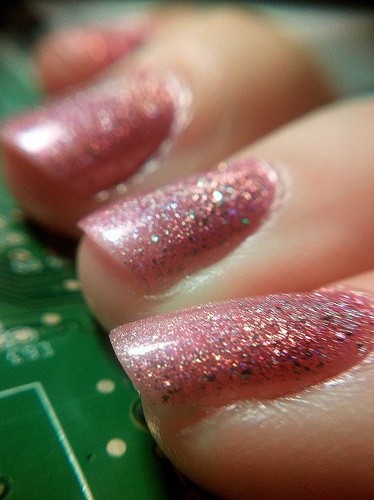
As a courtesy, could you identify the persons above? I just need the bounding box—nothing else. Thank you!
[1,7,372,500]
